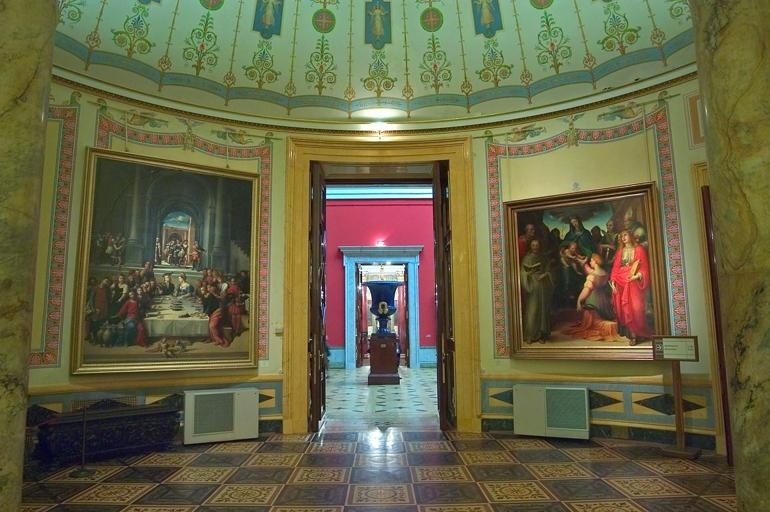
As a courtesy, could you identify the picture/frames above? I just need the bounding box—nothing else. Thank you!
[68,144,262,376]
[501,182,671,365]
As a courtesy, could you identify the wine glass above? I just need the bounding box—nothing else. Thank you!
[360,280,406,337]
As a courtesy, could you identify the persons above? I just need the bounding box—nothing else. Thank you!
[518,215,652,345]
[84,231,250,347]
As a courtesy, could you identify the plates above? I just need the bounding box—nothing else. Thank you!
[148,311,160,316]
[168,302,184,311]
